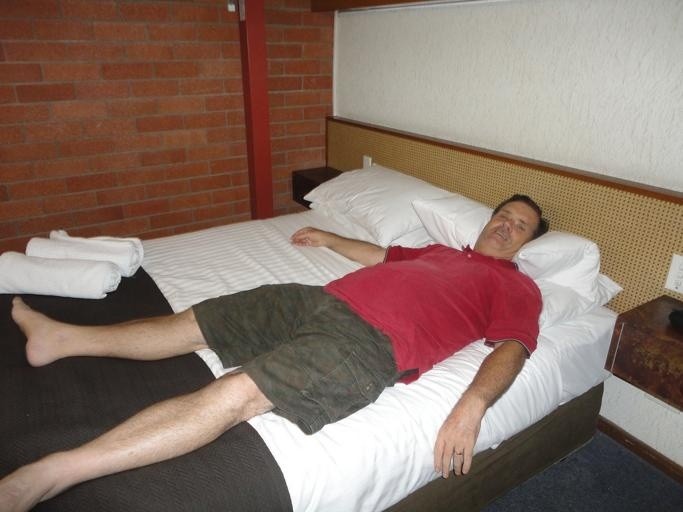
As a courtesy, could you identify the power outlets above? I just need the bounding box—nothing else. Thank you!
[362,156,372,168]
[664,253,683,295]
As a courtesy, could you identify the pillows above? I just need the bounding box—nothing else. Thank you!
[311,205,434,250]
[387,233,618,320]
[412,194,599,295]
[304,164,451,248]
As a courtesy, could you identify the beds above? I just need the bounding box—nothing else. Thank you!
[1,116,682,512]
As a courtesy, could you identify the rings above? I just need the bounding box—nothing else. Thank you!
[455,450,462,456]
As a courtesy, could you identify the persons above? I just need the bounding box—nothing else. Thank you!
[0,195,549,511]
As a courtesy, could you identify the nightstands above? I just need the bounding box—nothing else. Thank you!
[290,167,344,210]
[595,296,681,492]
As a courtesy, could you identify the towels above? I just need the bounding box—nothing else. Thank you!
[0,251,121,300]
[24,228,144,279]
